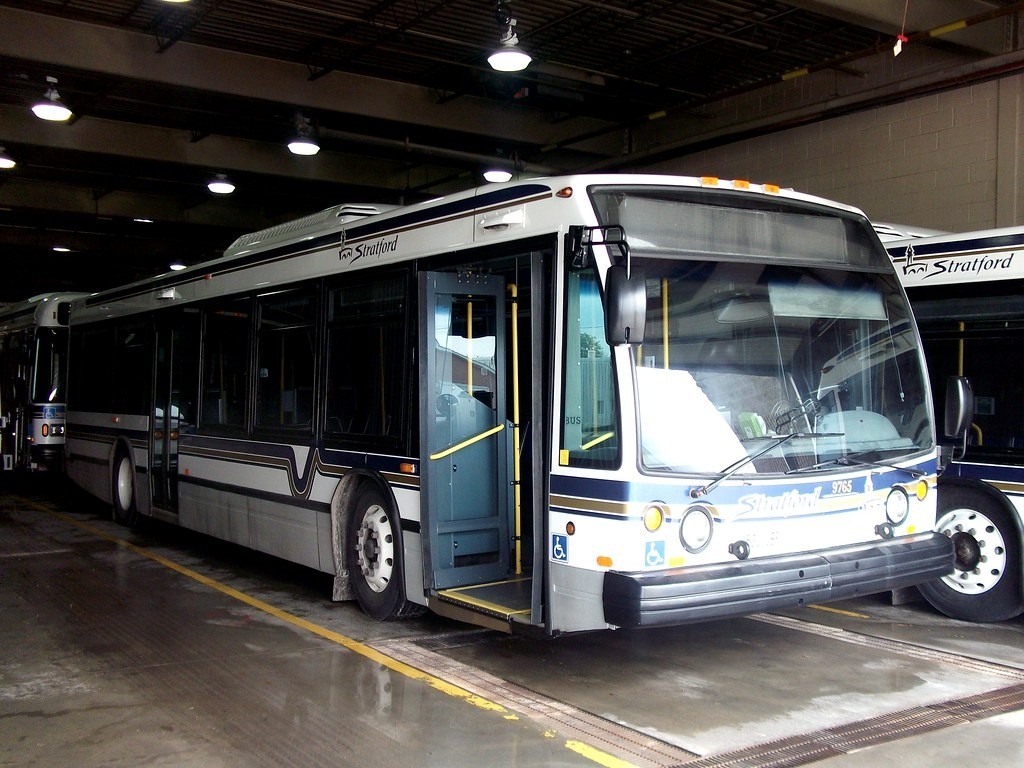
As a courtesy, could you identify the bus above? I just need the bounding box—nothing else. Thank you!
[855,222,1024,626]
[66,170,974,643]
[0,291,94,483]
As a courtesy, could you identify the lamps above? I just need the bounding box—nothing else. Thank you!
[207,170,235,194]
[31,77,72,121]
[487,12,532,72]
[285,117,320,155]
